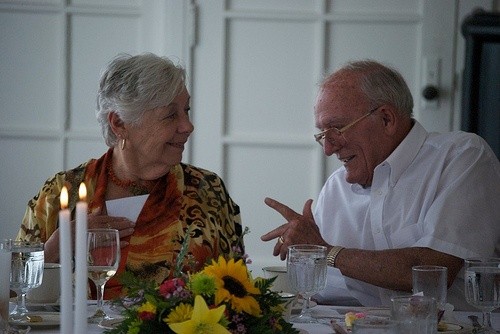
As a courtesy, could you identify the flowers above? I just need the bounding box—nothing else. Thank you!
[103,224,302,334]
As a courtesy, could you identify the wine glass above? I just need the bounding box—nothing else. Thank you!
[86,229,120,323]
[287,244,327,322]
[0,239,44,321]
[464,258,500,334]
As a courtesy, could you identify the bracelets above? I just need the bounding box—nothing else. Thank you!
[326,246,345,268]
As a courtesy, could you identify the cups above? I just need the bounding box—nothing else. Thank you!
[412,266,448,324]
[27,263,61,303]
[391,296,437,334]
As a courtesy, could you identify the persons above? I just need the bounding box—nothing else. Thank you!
[10,50,249,300]
[260,60,500,312]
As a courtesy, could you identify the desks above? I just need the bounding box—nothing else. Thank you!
[0,299,500,334]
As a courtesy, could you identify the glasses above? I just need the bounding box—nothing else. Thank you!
[314,105,382,148]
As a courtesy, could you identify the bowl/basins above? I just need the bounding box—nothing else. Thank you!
[262,266,289,291]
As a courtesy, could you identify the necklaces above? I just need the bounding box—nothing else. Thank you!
[107,159,150,196]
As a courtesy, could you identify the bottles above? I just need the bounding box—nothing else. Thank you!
[345,313,355,334]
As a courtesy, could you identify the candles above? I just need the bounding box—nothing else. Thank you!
[73,182,88,334]
[58,186,73,334]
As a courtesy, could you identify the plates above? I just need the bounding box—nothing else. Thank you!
[353,316,397,334]
[437,323,463,331]
[290,298,316,312]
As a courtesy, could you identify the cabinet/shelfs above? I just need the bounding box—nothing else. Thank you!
[461,10,500,159]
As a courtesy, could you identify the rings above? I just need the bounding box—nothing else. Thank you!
[104,223,112,240]
[279,236,284,244]
